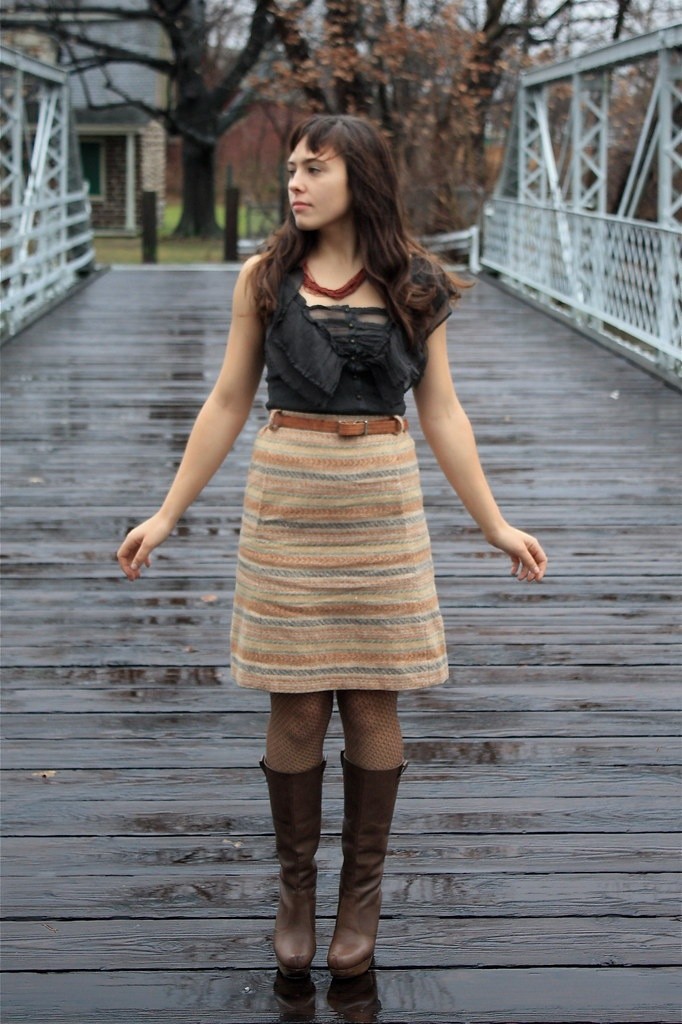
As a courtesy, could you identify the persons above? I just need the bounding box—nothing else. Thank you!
[118,116,548,980]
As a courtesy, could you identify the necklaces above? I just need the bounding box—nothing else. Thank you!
[301,267,366,300]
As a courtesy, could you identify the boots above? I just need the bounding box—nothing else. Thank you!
[259,753,329,981]
[326,747,410,978]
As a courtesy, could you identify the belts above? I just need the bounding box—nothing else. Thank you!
[272,413,407,437]
